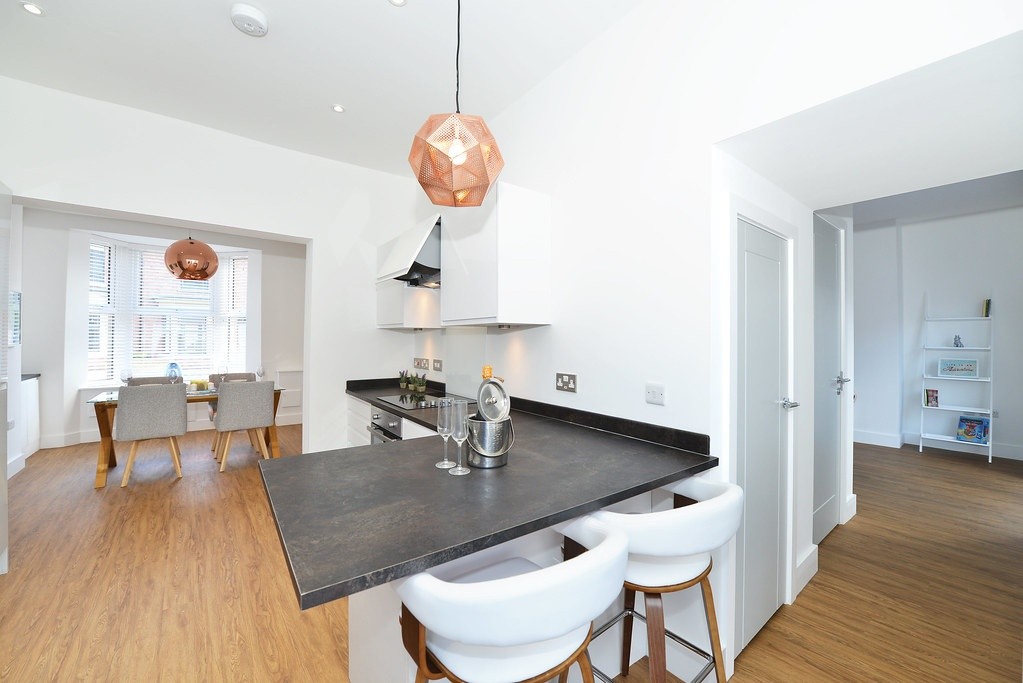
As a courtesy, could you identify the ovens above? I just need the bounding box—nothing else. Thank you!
[366,421,402,448]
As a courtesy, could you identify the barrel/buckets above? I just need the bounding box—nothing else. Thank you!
[464,377,516,470]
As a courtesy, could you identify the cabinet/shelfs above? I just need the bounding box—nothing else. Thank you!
[375,232,443,332]
[346,395,438,449]
[438,179,551,329]
[916,292,995,463]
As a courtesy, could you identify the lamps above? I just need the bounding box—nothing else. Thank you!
[409,2,504,206]
[164,227,219,281]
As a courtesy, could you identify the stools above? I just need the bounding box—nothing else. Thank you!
[386,524,631,683]
[546,475,743,683]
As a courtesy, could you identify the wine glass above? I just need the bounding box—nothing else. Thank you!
[435,394,456,469]
[119,368,132,386]
[448,403,471,476]
[256,366,265,381]
[218,365,228,382]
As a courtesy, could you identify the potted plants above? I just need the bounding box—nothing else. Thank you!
[398,369,428,392]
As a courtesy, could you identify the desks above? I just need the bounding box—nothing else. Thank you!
[84,383,284,488]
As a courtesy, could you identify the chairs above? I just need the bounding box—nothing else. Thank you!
[211,370,256,460]
[111,384,190,488]
[214,381,275,473]
[127,376,183,458]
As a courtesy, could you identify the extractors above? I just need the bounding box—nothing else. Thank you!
[394,225,441,286]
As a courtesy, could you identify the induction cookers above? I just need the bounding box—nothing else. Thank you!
[377,393,456,410]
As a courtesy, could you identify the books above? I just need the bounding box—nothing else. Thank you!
[956,415,989,444]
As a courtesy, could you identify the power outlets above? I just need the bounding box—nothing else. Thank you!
[413,358,442,371]
[555,372,576,393]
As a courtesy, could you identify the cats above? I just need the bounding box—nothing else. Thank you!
[954,335,964,346]
